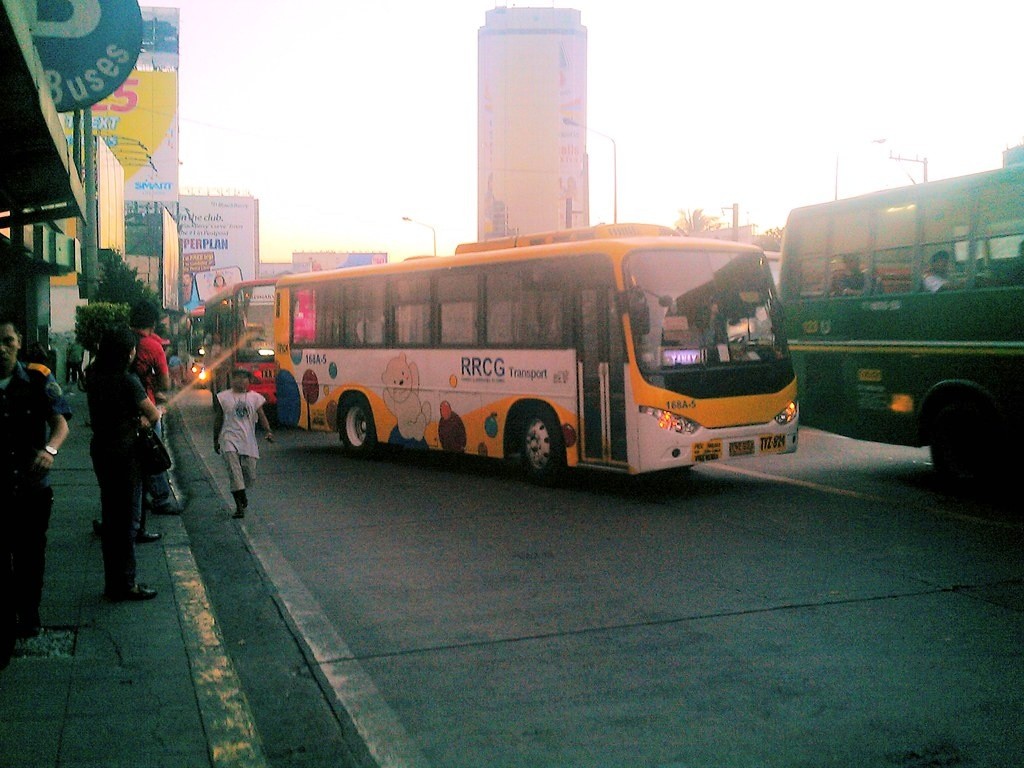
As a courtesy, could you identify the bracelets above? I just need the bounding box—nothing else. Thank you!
[45,445,58,456]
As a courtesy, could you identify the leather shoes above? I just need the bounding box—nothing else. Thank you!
[104,583,158,600]
[134,528,161,544]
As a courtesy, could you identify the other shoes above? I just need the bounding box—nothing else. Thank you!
[15,604,41,638]
[152,503,181,515]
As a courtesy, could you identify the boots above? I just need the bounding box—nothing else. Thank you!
[230,489,247,518]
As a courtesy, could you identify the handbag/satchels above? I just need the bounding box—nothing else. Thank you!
[141,427,172,476]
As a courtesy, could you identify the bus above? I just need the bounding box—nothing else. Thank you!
[174,279,315,413]
[778,162,1024,503]
[275,224,800,485]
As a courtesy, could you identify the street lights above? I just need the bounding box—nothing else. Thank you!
[563,119,616,224]
[402,217,436,259]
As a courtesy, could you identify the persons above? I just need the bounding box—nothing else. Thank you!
[920,251,965,294]
[0,301,184,674]
[830,253,885,296]
[213,367,275,518]
